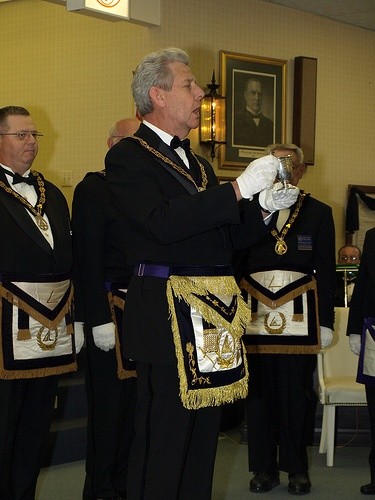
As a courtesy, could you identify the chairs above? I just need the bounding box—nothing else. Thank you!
[315,306,368,466]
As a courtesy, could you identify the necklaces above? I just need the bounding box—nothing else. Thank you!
[1,168,50,231]
[270,190,310,255]
[126,133,208,194]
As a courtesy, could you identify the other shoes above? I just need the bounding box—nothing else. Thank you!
[250,470,280,493]
[288,471,311,494]
[361,484,375,494]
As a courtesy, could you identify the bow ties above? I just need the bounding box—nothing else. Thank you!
[170,135,191,151]
[5,169,38,185]
[346,278,357,285]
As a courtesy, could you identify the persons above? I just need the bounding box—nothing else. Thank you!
[233,77,274,148]
[0,105,375,500]
[104,47,299,500]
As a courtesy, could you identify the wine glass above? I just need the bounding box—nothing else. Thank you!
[269,149,296,191]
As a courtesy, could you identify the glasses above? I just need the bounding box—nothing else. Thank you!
[340,256,359,261]
[0,131,44,140]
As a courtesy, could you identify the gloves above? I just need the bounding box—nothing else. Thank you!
[258,183,299,212]
[320,326,333,349]
[92,321,116,352]
[75,321,85,354]
[236,155,278,199]
[349,333,361,356]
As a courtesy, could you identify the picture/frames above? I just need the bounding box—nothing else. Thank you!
[217,49,288,172]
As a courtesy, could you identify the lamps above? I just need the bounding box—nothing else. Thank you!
[198,68,230,160]
